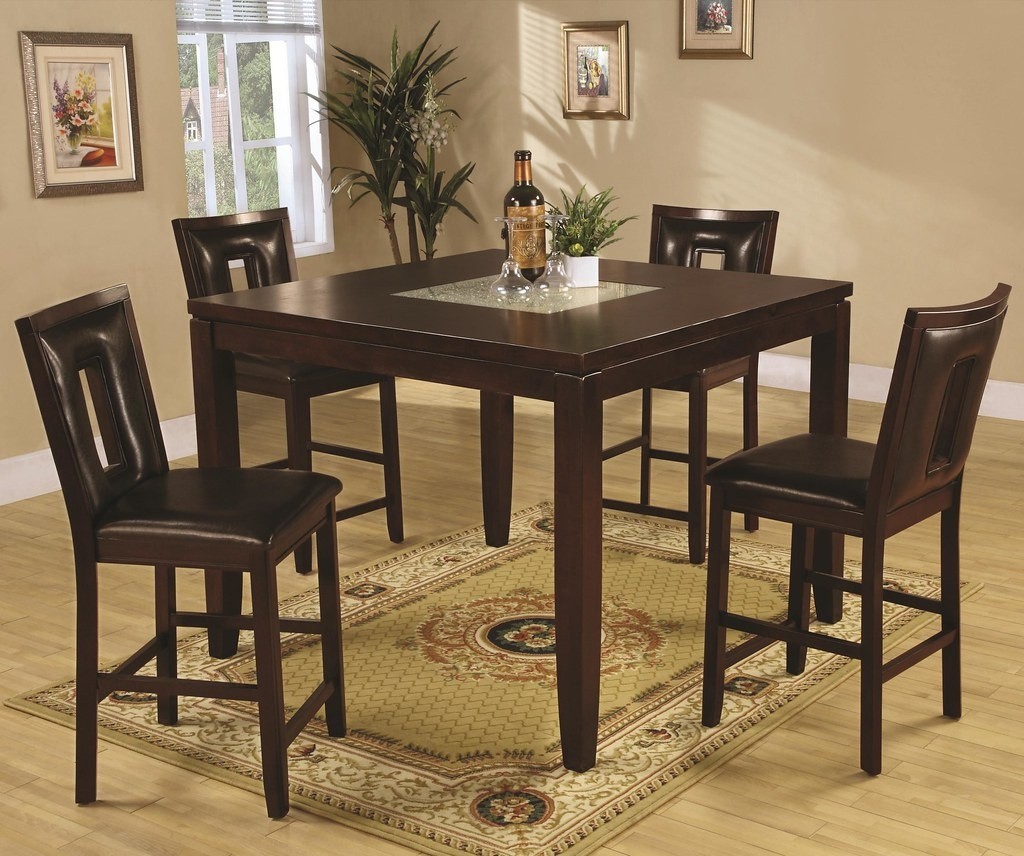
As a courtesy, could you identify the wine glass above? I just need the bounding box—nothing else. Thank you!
[534,215,574,306]
[489,217,535,307]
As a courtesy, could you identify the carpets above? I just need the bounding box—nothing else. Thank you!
[2,501,984,856]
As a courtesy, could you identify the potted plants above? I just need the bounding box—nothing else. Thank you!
[500,182,641,287]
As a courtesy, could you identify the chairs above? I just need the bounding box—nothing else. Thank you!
[703,282,1012,776]
[172,207,405,575]
[601,203,780,565]
[16,284,348,819]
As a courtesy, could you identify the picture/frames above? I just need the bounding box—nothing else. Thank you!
[679,0,754,60]
[18,30,145,199]
[560,20,631,121]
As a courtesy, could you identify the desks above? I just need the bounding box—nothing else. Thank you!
[184,248,853,773]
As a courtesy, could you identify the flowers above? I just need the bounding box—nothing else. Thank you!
[298,19,481,265]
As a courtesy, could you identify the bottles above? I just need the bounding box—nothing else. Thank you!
[505,150,546,281]
